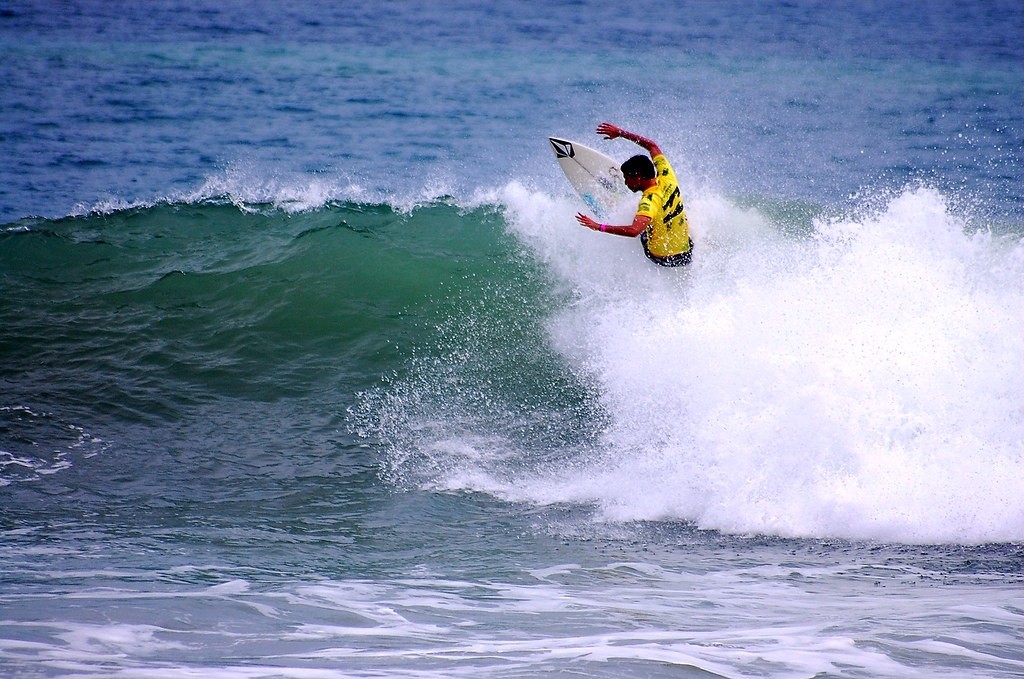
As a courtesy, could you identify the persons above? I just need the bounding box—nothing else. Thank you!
[575,122,695,268]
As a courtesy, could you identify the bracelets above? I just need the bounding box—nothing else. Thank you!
[601,225,605,232]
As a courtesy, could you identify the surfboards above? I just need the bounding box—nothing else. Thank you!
[546,134,690,318]
